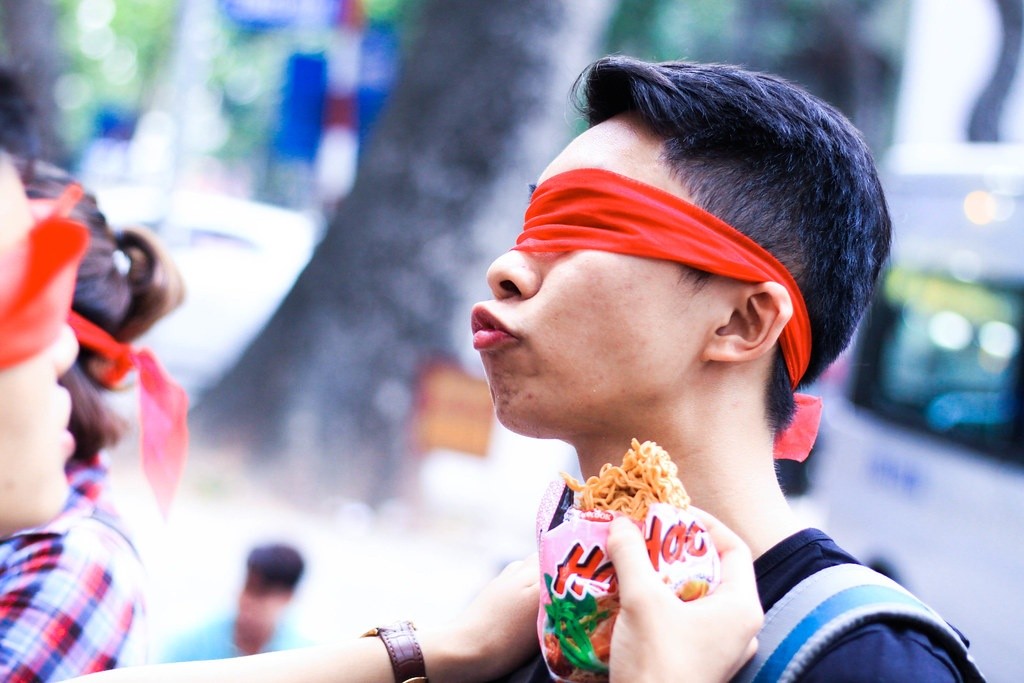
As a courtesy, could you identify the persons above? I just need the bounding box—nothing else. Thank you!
[0,68,764,683]
[469,55,987,683]
[165,542,305,666]
[0,157,185,683]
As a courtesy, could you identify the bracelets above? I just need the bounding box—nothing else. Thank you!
[360,620,429,683]
